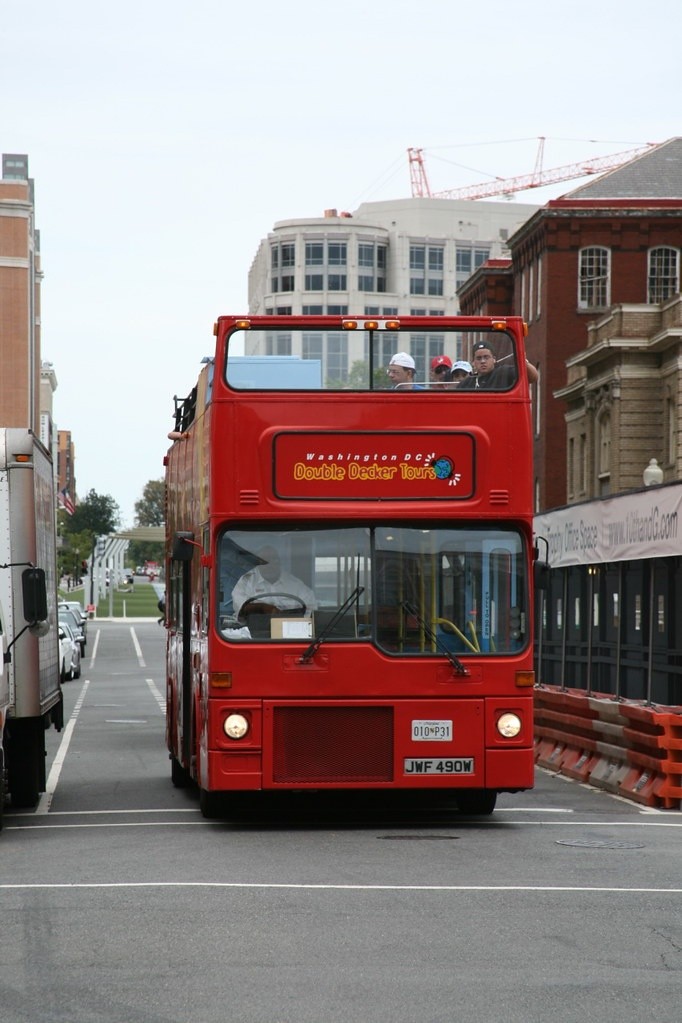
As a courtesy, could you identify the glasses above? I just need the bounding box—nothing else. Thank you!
[454,372,467,377]
[436,366,451,375]
[386,369,401,376]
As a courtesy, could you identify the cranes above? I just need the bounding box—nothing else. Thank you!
[401,135,660,204]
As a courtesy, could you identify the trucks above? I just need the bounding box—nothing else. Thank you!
[0,428,65,808]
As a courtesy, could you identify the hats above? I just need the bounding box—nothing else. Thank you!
[431,355,452,372]
[450,361,473,376]
[472,341,497,358]
[389,352,419,377]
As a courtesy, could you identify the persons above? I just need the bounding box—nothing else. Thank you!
[386,352,427,390]
[429,355,452,389]
[457,341,538,389]
[231,545,318,616]
[442,360,474,390]
[157,590,166,626]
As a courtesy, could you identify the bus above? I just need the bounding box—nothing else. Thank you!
[154,310,554,822]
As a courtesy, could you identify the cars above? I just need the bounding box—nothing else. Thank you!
[54,600,95,683]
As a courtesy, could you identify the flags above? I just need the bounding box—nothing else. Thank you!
[57,487,75,516]
